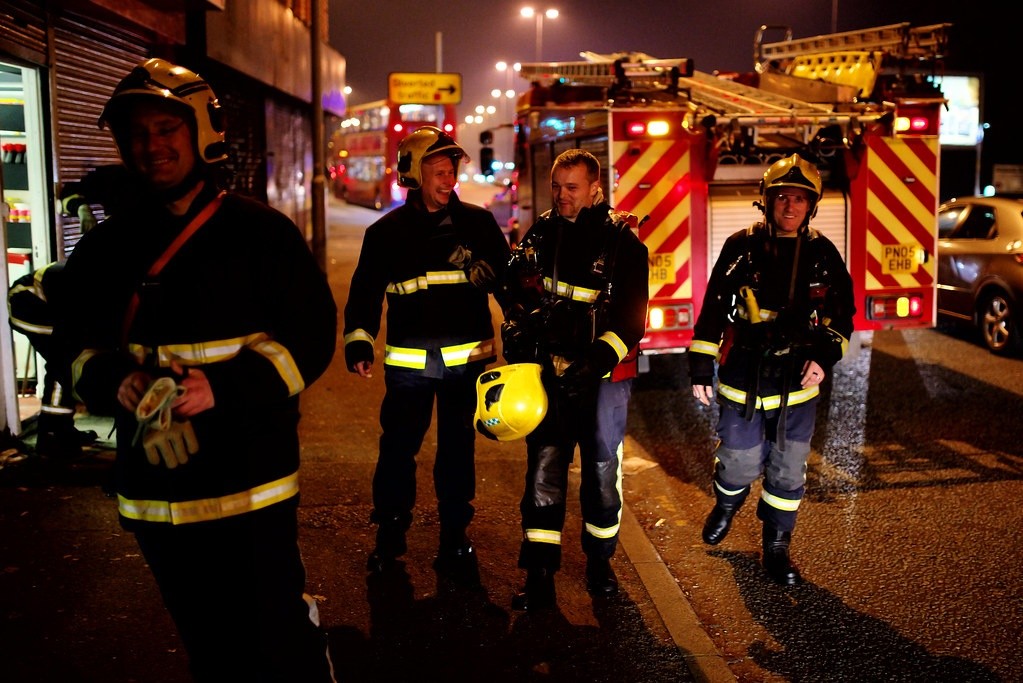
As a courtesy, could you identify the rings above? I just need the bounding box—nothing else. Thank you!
[812,372,818,376]
[817,376,820,380]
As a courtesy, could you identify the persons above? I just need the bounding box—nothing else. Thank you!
[64,162,124,233]
[689,152,857,584]
[499,147,650,612]
[55,58,337,683]
[343,123,511,570]
[8,259,68,452]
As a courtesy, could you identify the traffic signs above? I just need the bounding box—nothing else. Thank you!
[387,70,463,106]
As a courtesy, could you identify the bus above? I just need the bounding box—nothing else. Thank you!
[327,99,457,211]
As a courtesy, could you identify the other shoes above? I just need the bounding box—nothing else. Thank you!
[38,421,80,461]
[37,410,98,441]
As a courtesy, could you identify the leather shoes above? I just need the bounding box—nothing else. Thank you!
[367,520,407,568]
[584,557,619,597]
[763,523,801,586]
[511,572,556,612]
[439,512,475,558]
[702,494,745,545]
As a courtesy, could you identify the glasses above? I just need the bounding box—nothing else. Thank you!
[772,194,810,209]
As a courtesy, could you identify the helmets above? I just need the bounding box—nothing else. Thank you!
[472,361,549,442]
[397,126,471,188]
[96,56,232,176]
[759,151,825,218]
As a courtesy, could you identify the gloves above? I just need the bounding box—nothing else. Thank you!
[134,377,198,468]
[78,204,98,234]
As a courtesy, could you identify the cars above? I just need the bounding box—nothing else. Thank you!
[936,194,1023,355]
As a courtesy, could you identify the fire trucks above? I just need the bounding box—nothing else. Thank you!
[475,14,958,362]
